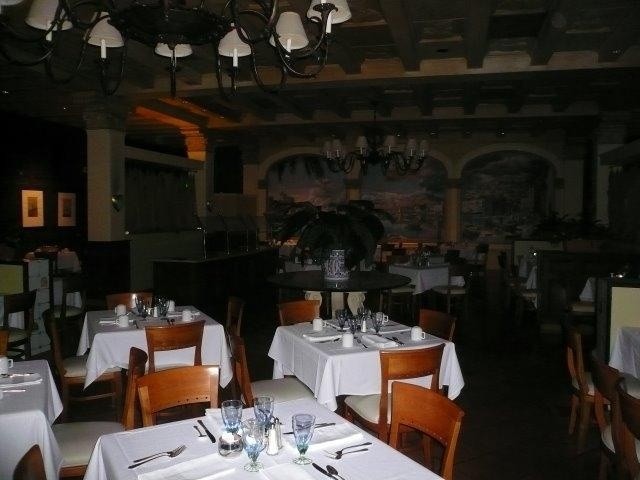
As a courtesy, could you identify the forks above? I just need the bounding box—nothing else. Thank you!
[324,441,373,460]
[127,444,187,469]
[168,318,175,329]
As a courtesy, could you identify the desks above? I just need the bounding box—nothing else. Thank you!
[97,396,442,480]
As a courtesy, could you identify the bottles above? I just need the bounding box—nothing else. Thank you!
[409,249,432,269]
[266,418,285,457]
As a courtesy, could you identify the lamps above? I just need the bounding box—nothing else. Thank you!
[0,0,353,101]
[320,110,431,177]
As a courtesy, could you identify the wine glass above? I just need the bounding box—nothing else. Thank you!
[133,295,168,321]
[334,308,381,336]
[221,395,274,471]
[291,412,314,466]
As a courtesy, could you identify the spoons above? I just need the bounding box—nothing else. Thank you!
[192,425,206,438]
[326,464,346,480]
[355,337,367,350]
[134,320,140,331]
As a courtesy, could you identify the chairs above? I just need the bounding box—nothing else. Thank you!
[510,265,640,480]
[343,344,446,474]
[0,242,490,431]
[390,382,465,478]
[24,345,149,478]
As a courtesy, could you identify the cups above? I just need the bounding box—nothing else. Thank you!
[115,302,130,327]
[411,326,425,342]
[167,299,196,324]
[0,356,14,373]
[313,317,327,332]
[377,311,390,326]
[342,331,354,348]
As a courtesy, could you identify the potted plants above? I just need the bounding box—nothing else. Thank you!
[262,198,396,281]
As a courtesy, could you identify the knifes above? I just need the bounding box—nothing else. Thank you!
[6,372,33,377]
[385,335,406,347]
[312,462,336,480]
[198,418,216,444]
[1,389,26,393]
[283,422,337,435]
[313,337,340,345]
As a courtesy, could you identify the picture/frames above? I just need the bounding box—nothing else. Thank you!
[19,186,78,231]
